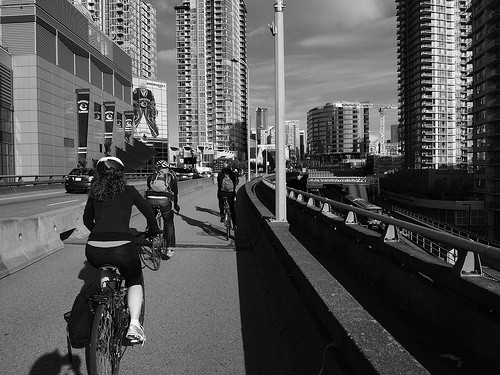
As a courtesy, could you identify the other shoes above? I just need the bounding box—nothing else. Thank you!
[166,249,174,256]
[220,216,224,222]
[233,225,237,230]
[126,324,147,347]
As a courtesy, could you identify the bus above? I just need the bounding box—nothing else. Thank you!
[342,194,383,223]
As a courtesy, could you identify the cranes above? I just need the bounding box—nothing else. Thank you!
[333,102,399,155]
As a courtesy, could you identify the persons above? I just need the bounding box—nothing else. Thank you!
[217,162,238,230]
[145,159,180,256]
[82,156,160,344]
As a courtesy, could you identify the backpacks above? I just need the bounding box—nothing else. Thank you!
[221,173,234,192]
[150,169,171,192]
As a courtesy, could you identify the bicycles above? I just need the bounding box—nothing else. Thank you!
[143,193,179,271]
[217,190,237,241]
[85,231,164,375]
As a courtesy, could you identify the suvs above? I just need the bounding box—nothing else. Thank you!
[65,168,98,194]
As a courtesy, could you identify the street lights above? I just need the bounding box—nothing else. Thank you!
[462,203,472,239]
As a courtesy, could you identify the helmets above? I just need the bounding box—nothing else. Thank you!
[97,156,125,175]
[155,160,169,169]
